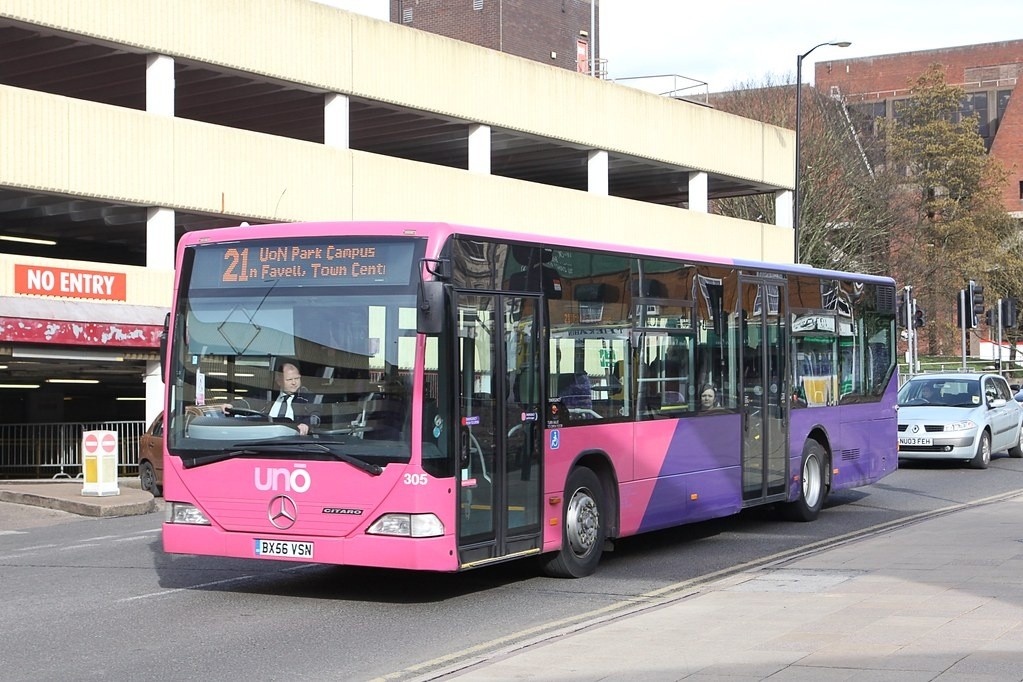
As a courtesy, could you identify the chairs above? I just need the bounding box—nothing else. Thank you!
[559,342,886,419]
[921,387,944,405]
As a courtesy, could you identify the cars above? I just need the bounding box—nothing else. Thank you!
[1009,383,1023,403]
[897,371,1023,470]
[138,406,232,498]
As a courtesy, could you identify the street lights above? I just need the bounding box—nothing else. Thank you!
[794,41,856,260]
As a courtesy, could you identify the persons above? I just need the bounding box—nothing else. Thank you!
[221,363,321,435]
[694,328,755,379]
[513,342,561,481]
[911,385,941,403]
[649,344,681,393]
[555,345,593,421]
[695,383,718,414]
[607,339,652,418]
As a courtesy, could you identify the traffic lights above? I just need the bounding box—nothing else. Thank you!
[916,307,927,327]
[968,280,985,328]
[984,309,996,327]
[898,303,909,327]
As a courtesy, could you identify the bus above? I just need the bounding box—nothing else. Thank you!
[155,219,898,579]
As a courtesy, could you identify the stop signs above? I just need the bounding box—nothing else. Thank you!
[83,430,118,456]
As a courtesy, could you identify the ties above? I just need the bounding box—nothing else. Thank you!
[278,394,291,418]
